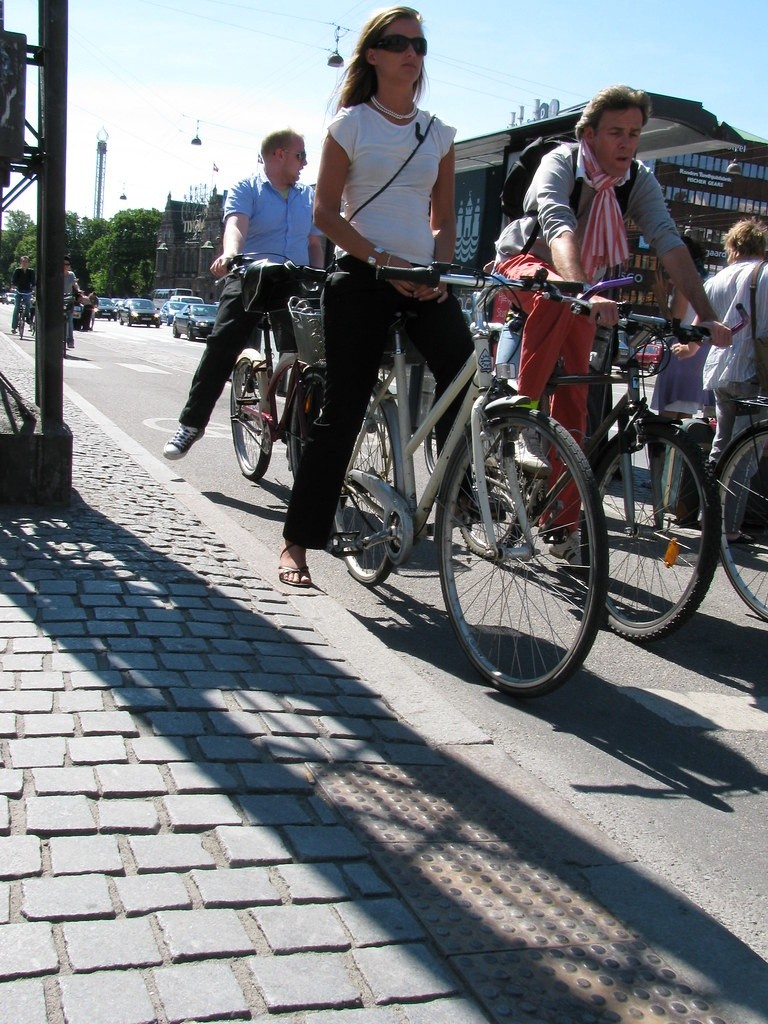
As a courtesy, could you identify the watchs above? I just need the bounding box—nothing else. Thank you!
[571,283,591,297]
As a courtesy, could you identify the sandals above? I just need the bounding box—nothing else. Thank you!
[447,493,506,520]
[278,542,312,588]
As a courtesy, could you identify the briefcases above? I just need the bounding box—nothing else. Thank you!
[659,416,768,528]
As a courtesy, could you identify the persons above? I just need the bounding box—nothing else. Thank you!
[76,292,99,332]
[491,86,734,567]
[672,218,768,544]
[278,6,507,587]
[11,256,36,333]
[642,240,711,489]
[163,131,324,461]
[64,261,83,348]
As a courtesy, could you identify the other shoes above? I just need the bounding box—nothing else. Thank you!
[67,341,74,348]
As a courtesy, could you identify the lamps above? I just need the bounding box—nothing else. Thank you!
[156,242,169,251]
[201,239,215,249]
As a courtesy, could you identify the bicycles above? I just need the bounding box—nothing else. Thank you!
[451,271,725,646]
[281,249,612,700]
[211,250,768,622]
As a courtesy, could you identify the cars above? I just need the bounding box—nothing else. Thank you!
[0,287,206,359]
[171,303,219,341]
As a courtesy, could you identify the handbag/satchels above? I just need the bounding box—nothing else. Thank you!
[756,338,768,391]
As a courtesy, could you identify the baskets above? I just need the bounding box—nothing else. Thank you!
[289,310,326,368]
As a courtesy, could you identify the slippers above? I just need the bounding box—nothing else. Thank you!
[727,533,755,544]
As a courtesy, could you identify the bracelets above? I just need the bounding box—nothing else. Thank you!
[385,254,392,282]
[368,246,385,265]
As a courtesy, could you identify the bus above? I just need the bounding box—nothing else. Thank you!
[149,287,193,313]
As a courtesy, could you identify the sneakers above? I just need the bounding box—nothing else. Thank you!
[163,425,204,460]
[509,428,554,476]
[548,531,583,568]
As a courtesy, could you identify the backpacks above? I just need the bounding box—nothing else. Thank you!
[499,133,640,220]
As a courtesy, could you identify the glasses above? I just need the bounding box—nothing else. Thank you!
[370,34,428,56]
[283,151,307,162]
[64,263,71,266]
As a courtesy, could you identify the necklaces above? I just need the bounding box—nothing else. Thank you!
[371,96,417,119]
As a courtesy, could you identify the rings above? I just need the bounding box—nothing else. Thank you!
[433,287,438,292]
[438,290,442,295]
